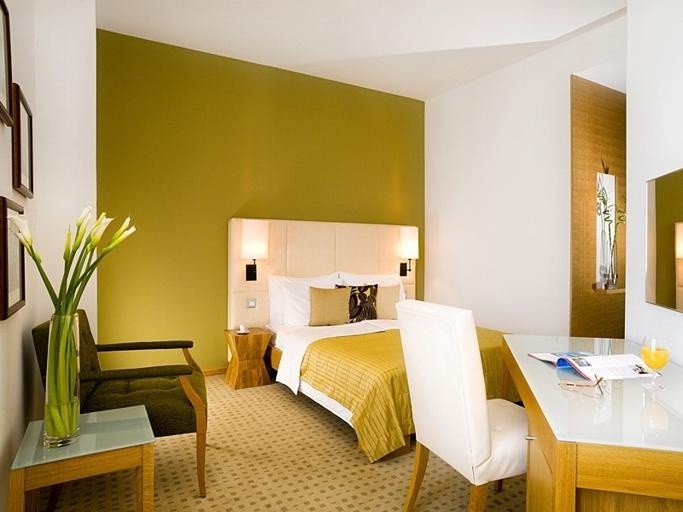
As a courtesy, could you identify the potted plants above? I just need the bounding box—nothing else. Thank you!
[597,194,627,290]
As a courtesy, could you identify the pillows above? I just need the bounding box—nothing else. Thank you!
[267,272,408,326]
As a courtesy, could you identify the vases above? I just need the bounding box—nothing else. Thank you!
[41,313,81,450]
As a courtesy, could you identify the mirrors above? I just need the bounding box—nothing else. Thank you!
[645,166,683,314]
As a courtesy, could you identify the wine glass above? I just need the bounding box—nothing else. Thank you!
[641,337,671,389]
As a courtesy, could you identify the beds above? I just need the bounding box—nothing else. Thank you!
[226,217,521,464]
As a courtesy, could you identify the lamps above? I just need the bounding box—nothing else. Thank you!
[239,218,270,269]
[398,225,420,271]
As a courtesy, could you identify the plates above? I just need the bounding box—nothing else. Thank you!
[237,331,249,334]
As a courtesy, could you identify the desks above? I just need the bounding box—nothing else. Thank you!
[500,332,683,512]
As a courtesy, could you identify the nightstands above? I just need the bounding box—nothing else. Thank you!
[223,327,274,391]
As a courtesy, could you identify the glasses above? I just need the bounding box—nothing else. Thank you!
[559,374,604,398]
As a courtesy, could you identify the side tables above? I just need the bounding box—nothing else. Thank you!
[7,405,157,512]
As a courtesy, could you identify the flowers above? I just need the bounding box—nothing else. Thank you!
[7,205,137,441]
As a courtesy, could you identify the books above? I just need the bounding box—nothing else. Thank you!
[528,351,662,382]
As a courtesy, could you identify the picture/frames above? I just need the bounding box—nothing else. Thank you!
[0,1,34,320]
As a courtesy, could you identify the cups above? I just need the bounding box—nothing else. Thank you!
[240,325,247,332]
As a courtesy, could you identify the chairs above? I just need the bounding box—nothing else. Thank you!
[396,298,529,512]
[31,308,208,499]
